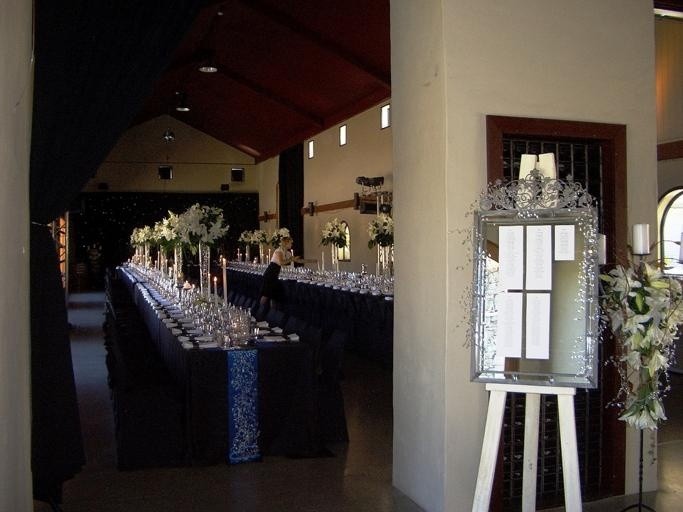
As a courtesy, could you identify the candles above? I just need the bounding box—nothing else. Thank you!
[632,221,650,255]
[594,233,606,264]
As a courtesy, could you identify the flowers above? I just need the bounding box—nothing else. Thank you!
[128,204,227,252]
[238,215,394,249]
[600,237,682,432]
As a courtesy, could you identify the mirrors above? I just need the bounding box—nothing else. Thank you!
[461,165,606,388]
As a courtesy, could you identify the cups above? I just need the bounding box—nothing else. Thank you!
[227,261,393,295]
[131,262,258,348]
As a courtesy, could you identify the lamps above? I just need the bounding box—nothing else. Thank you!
[160,43,217,114]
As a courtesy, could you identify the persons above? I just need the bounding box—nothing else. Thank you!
[256,235,318,311]
